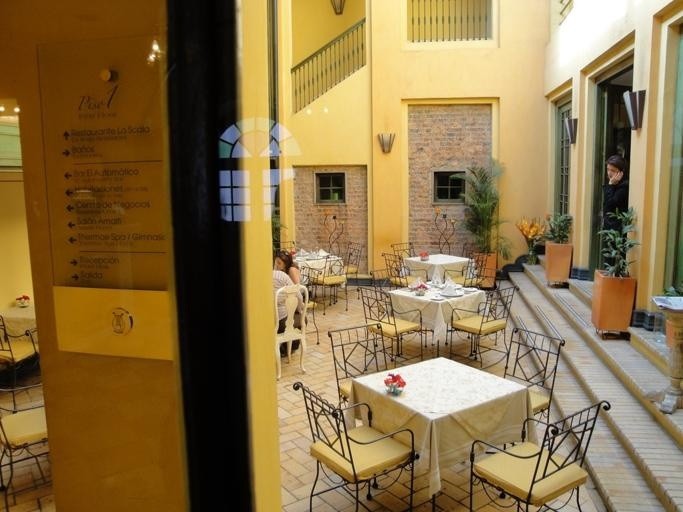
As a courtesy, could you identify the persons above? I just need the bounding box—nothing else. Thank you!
[274,270,307,355]
[275,250,300,285]
[603,155,629,269]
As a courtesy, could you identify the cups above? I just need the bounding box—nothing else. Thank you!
[332,193,337,200]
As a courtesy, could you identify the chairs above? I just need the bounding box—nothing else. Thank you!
[0,315,42,414]
[0,384,52,512]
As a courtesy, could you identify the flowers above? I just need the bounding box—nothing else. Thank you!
[16,295,31,301]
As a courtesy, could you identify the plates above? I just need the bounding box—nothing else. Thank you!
[412,280,477,301]
[296,254,343,263]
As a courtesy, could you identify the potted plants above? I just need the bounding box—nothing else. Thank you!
[591,207,642,339]
[541,213,573,287]
[514,215,546,265]
[450,158,512,290]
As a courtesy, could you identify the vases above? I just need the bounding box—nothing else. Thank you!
[16,301,30,307]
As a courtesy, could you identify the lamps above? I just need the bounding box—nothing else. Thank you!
[330,0,345,15]
[377,132,396,153]
